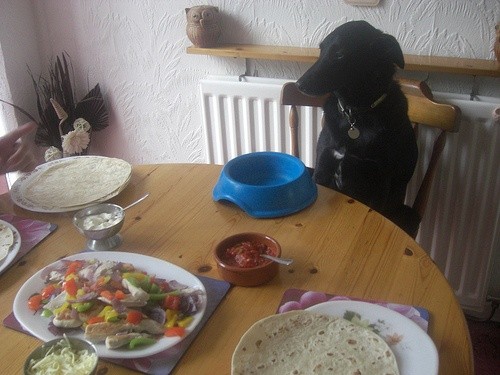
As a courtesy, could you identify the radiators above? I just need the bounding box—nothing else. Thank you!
[203,75,499,322]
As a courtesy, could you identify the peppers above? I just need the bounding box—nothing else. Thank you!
[29,260,196,349]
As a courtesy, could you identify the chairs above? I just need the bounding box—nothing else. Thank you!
[281,78,461,240]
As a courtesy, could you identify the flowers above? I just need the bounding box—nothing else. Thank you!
[1,51,113,163]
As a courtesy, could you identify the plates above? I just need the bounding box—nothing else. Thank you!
[10,155,129,213]
[12,250,207,358]
[0,218,22,271]
[305,300,440,374]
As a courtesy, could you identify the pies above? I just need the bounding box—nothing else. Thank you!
[0,222,13,261]
[22,156,132,208]
[230,310,400,375]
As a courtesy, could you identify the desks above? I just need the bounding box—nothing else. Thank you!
[0,161,474,375]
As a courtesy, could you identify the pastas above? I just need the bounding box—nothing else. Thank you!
[26,332,95,375]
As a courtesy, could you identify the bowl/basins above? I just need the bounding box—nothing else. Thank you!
[212,152,319,219]
[23,337,98,375]
[213,232,282,286]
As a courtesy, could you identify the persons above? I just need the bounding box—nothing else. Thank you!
[0,122,37,174]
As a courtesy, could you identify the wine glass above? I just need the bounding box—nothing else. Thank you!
[72,203,126,252]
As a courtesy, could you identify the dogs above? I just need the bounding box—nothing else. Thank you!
[295,19,423,239]
